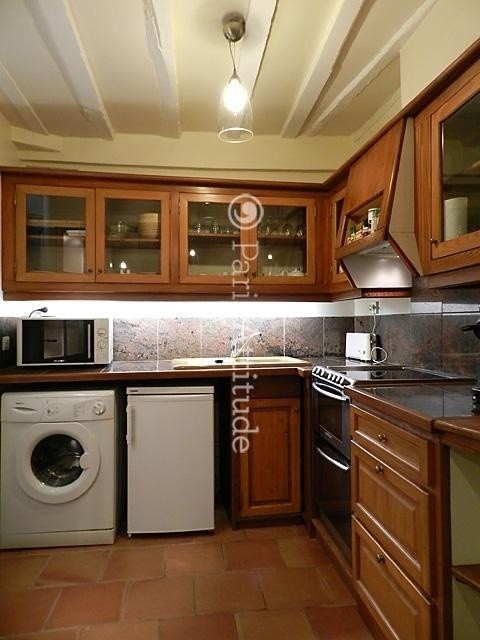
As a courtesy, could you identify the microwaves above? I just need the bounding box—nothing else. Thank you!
[15,316,114,368]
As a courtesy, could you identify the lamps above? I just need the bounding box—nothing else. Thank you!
[218,12,254,145]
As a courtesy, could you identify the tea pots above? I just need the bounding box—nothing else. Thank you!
[287,269,306,276]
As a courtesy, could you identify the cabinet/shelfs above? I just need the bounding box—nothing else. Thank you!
[413,52,480,275]
[323,178,349,285]
[172,176,322,302]
[347,397,436,640]
[436,424,480,640]
[1,166,171,300]
[232,370,302,525]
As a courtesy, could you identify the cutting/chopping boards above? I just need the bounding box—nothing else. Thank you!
[170,354,309,371]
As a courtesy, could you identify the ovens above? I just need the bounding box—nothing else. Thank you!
[310,364,448,570]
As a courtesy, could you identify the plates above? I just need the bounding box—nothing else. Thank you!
[138,212,161,239]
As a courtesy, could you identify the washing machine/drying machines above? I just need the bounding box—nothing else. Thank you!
[0,391,115,547]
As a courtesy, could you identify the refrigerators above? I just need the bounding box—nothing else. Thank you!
[125,387,216,540]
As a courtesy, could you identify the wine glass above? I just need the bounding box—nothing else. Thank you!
[294,214,306,237]
[282,213,294,235]
[263,214,273,235]
